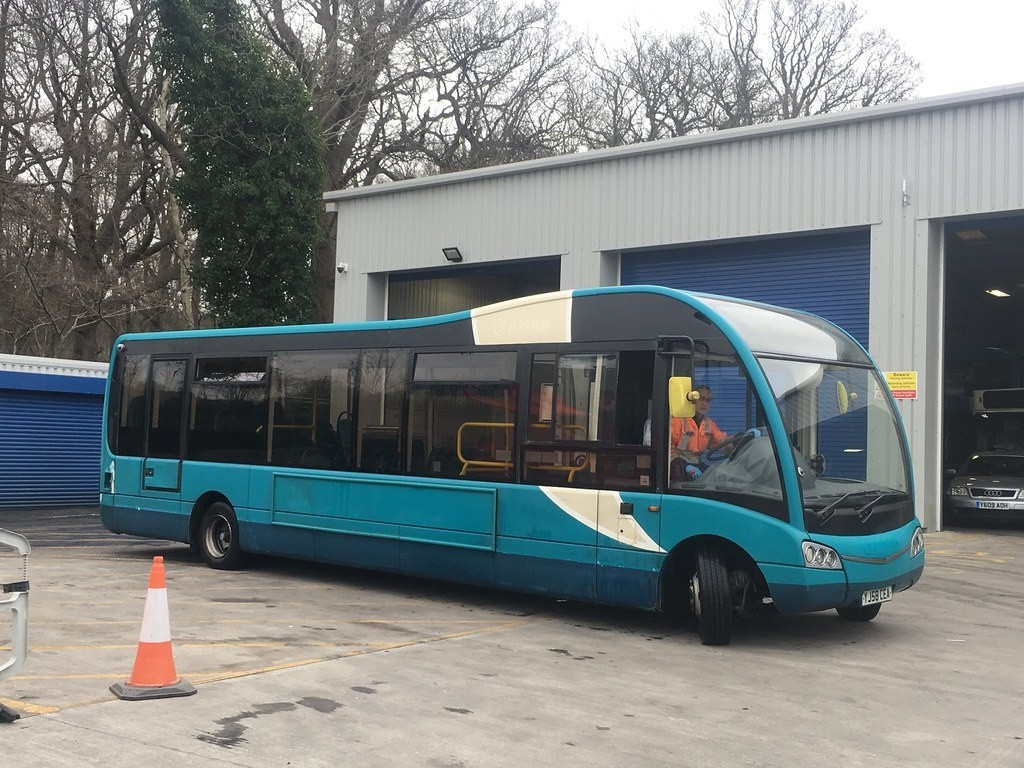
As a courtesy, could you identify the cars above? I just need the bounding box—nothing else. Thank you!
[945,451,1024,527]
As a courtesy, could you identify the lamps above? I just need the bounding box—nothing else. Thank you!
[442,247,462,263]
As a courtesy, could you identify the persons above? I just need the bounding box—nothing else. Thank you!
[670,384,764,481]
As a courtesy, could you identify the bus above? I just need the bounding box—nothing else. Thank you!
[97,284,926,647]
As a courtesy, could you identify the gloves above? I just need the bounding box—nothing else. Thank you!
[746,428,762,438]
[685,464,702,479]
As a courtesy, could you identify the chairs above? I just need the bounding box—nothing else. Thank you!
[982,464,993,471]
[127,393,456,479]
[643,400,651,445]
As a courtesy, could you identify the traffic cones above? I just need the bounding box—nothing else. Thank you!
[110,555,199,702]
